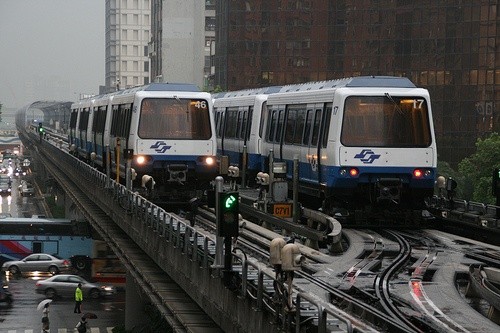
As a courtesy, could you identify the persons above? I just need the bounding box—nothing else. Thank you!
[74,284,83,313]
[39,303,52,333]
[75,316,88,333]
[446,176,457,200]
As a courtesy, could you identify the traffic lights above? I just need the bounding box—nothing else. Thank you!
[224,195,237,210]
[39,128,43,132]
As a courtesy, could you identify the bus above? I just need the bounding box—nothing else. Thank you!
[0,214,99,269]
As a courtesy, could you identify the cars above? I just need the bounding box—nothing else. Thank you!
[34,274,115,300]
[2,253,72,276]
[0,152,34,196]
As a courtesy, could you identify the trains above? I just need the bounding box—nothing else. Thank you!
[69,83,217,199]
[212,76,437,218]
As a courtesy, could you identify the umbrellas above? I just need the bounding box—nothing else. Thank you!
[80,312,97,319]
[36,299,52,310]
